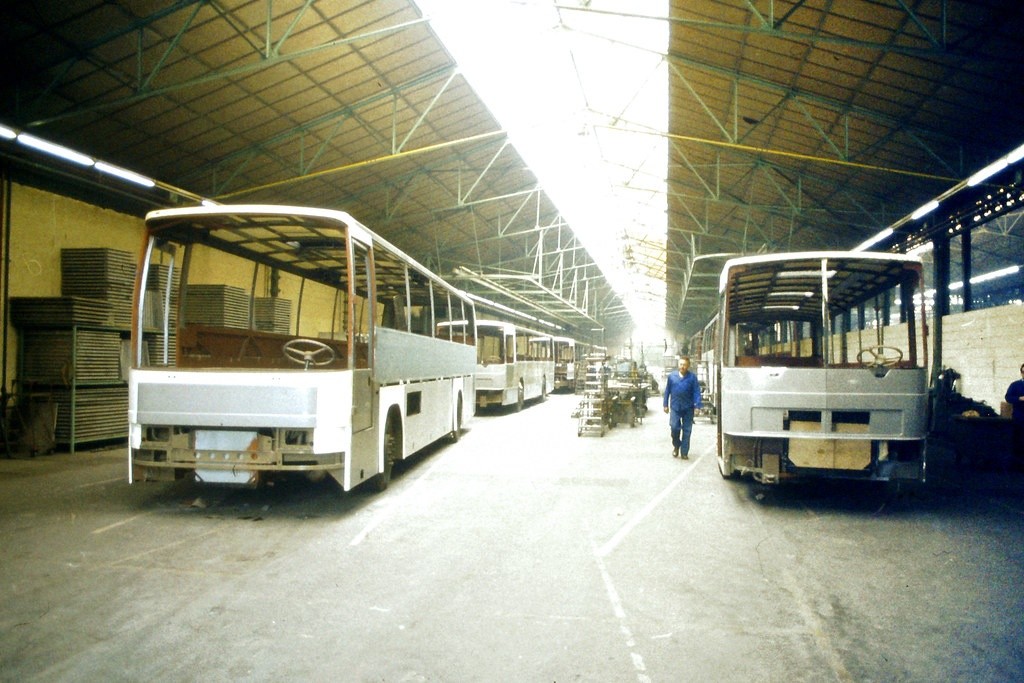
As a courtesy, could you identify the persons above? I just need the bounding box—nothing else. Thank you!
[1003,364,1024,446]
[662,355,704,459]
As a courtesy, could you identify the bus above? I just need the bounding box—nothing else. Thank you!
[716,250,929,501]
[434,318,555,411]
[527,336,607,390]
[686,315,714,412]
[125,203,478,493]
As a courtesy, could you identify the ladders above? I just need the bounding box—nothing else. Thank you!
[576,326,612,436]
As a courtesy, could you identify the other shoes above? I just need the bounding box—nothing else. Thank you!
[673,447,679,457]
[681,454,688,459]
